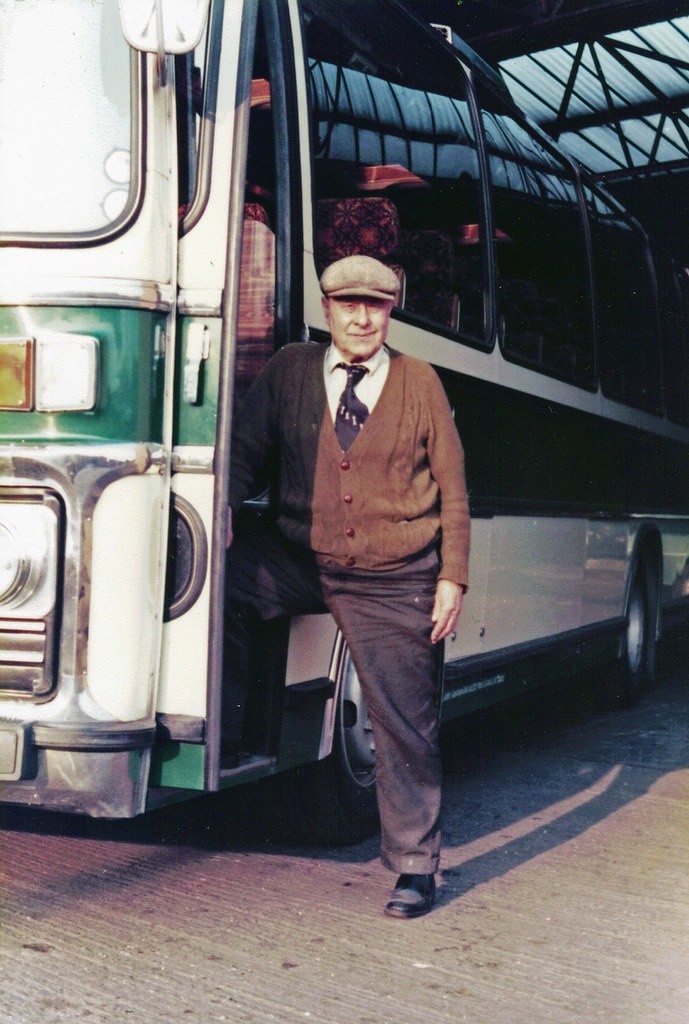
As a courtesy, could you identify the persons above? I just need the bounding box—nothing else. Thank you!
[219,254,470,916]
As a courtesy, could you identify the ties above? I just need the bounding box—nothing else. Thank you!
[334,365,368,454]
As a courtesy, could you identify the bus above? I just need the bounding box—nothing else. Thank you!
[0,0,689,817]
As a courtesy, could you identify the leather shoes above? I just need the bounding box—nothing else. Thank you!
[385,871,435,917]
[218,739,240,769]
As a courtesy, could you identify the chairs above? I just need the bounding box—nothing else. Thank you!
[178,194,630,400]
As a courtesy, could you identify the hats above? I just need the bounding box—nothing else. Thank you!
[320,254,400,301]
